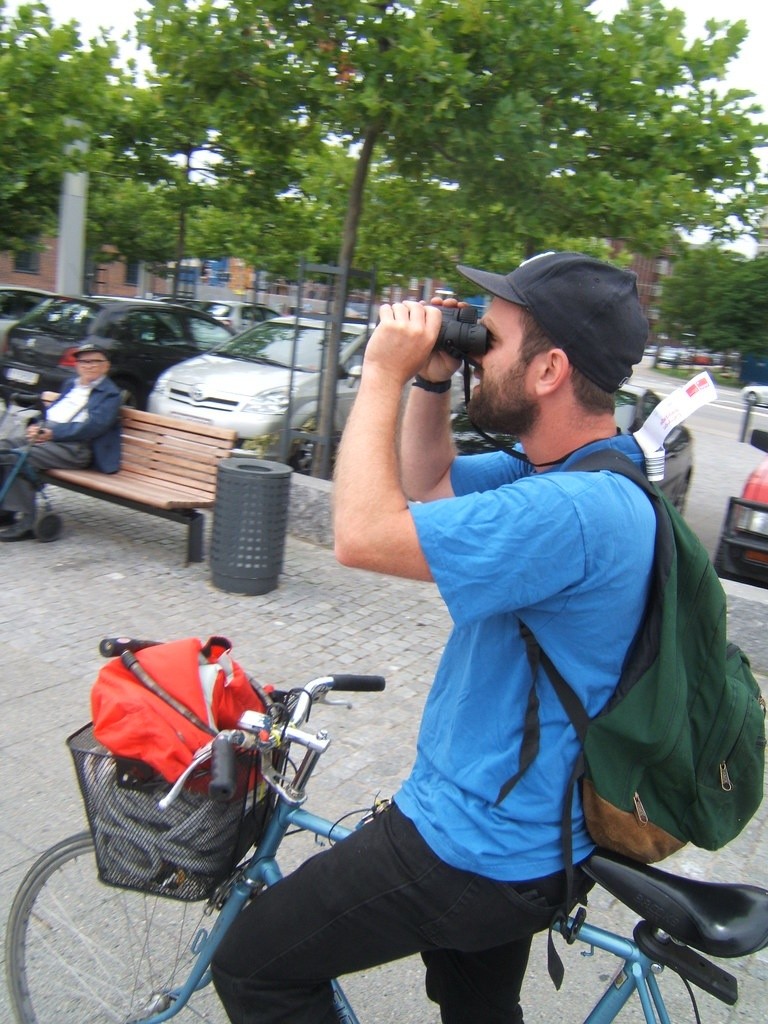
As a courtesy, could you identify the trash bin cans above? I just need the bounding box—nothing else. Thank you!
[205,456,293,593]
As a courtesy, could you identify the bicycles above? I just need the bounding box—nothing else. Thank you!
[1,634,767,1024]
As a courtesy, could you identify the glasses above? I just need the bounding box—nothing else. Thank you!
[74,358,106,365]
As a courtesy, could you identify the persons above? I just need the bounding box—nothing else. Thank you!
[0,342,122,542]
[211,249,765,1024]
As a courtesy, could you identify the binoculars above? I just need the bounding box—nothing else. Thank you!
[371,300,494,359]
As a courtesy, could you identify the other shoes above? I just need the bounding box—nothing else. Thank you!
[0,512,36,542]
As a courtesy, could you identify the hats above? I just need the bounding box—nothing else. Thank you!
[71,343,111,356]
[457,250,647,393]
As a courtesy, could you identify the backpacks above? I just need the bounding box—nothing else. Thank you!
[494,448,767,991]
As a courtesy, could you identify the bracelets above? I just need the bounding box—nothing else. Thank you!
[411,373,452,394]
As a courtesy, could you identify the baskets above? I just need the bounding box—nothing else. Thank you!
[65,721,291,902]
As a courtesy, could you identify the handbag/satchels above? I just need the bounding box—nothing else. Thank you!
[91,637,275,810]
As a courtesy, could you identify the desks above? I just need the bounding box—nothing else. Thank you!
[32,390,239,563]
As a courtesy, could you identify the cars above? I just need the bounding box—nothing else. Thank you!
[450,380,696,520]
[710,427,768,585]
[644,346,740,367]
[178,301,282,351]
[148,314,482,477]
[131,293,202,312]
[0,284,55,362]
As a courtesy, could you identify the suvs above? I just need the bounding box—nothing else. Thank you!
[1,294,243,418]
